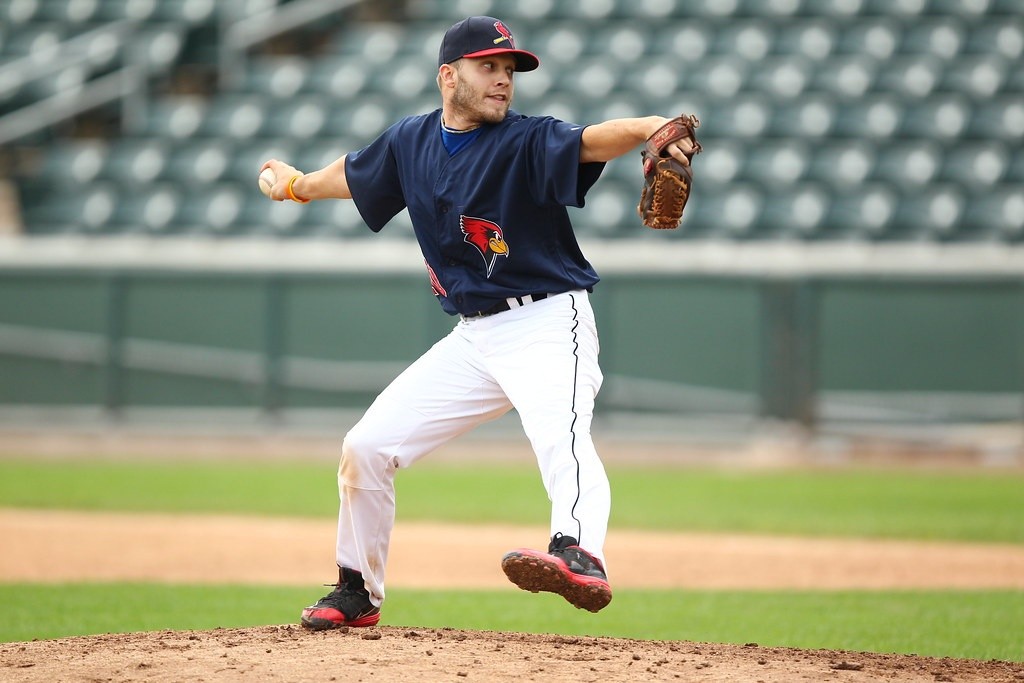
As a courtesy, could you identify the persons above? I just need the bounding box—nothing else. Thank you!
[253,16,692,627]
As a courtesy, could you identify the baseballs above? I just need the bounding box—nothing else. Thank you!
[258,168,277,197]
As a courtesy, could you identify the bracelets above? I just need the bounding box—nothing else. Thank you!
[287,175,312,204]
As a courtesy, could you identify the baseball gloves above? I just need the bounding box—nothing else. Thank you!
[637,111,705,231]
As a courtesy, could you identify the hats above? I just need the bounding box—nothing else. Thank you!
[438,15,540,73]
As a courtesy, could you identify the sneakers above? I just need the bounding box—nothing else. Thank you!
[502,532,612,614]
[302,563,380,628]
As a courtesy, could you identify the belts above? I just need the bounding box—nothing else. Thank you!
[463,291,548,317]
